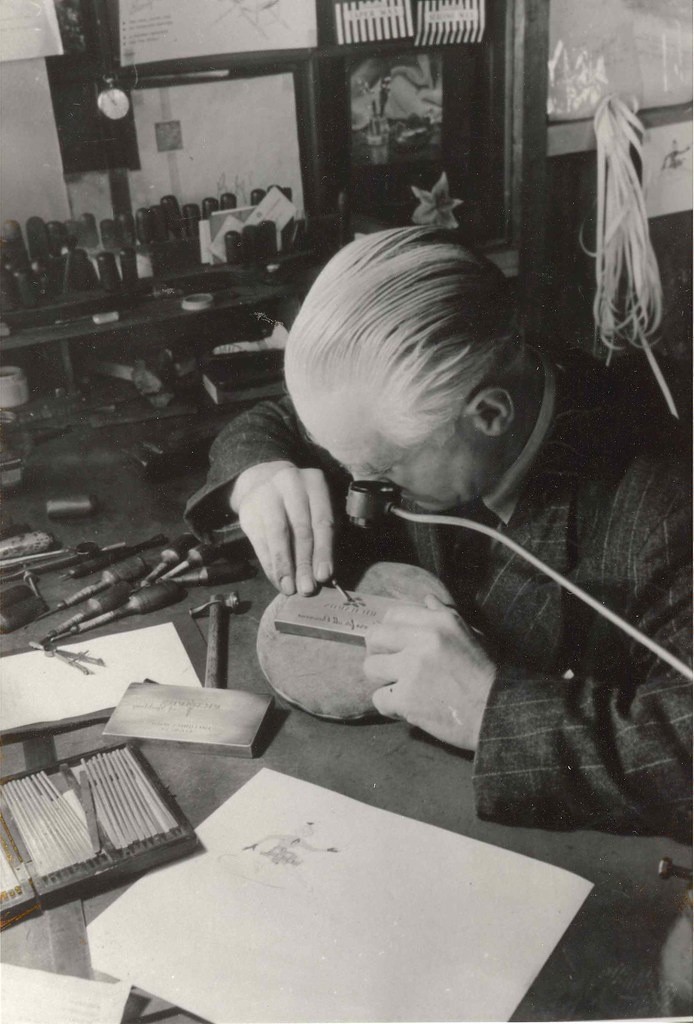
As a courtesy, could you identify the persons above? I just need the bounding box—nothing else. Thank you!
[184,224,693,845]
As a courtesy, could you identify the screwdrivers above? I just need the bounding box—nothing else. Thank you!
[32,530,255,642]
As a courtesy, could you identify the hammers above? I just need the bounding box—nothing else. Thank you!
[188,591,241,689]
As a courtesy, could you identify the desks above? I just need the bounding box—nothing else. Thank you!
[0,390,694,1024]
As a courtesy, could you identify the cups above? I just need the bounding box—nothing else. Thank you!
[0,365,30,409]
